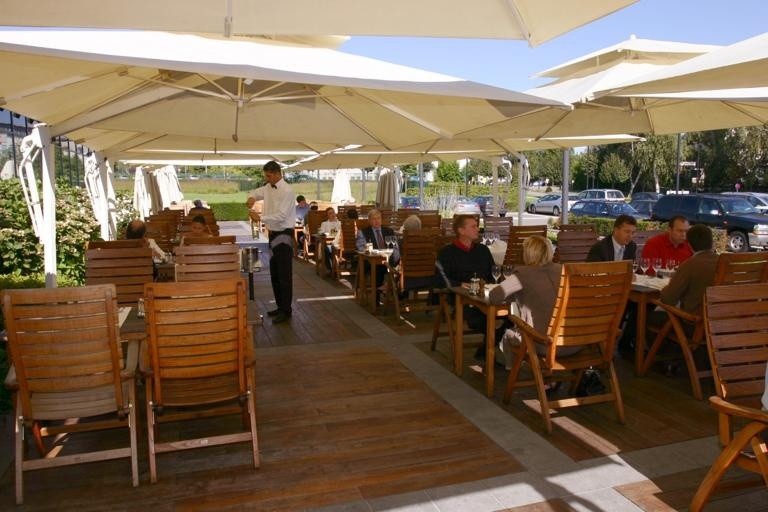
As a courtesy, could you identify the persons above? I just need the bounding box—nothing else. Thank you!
[357,210,423,305]
[434,214,515,368]
[760,364,768,446]
[488,235,586,400]
[586,214,733,394]
[246,161,296,324]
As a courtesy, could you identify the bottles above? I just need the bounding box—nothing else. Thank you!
[364,242,378,255]
[138,297,146,319]
[252,210,259,240]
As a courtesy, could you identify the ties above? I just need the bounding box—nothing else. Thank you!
[376,230,385,249]
[615,247,625,261]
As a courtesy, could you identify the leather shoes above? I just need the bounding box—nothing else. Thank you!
[617,340,639,360]
[267,308,292,317]
[272,314,290,325]
[544,381,561,398]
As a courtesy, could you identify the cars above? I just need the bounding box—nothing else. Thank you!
[473,195,507,218]
[401,196,429,210]
[116,171,317,183]
[570,189,768,252]
[404,174,427,183]
[436,198,481,216]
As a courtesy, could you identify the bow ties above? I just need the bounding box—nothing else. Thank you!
[270,184,278,189]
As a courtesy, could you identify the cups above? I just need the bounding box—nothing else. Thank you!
[316,226,338,237]
[153,251,176,264]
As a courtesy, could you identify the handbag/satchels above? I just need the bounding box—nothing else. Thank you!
[576,369,606,397]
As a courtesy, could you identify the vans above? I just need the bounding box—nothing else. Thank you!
[527,192,584,215]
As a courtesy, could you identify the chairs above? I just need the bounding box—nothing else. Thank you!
[246,195,266,239]
[641,251,768,401]
[294,204,444,326]
[143,200,221,252]
[430,214,598,356]
[1,284,140,504]
[687,282,768,512]
[139,278,260,484]
[86,235,242,303]
[634,230,662,259]
[504,259,634,435]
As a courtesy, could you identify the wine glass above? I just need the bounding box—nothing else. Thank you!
[482,232,501,248]
[632,258,680,282]
[383,236,397,252]
[467,264,514,297]
